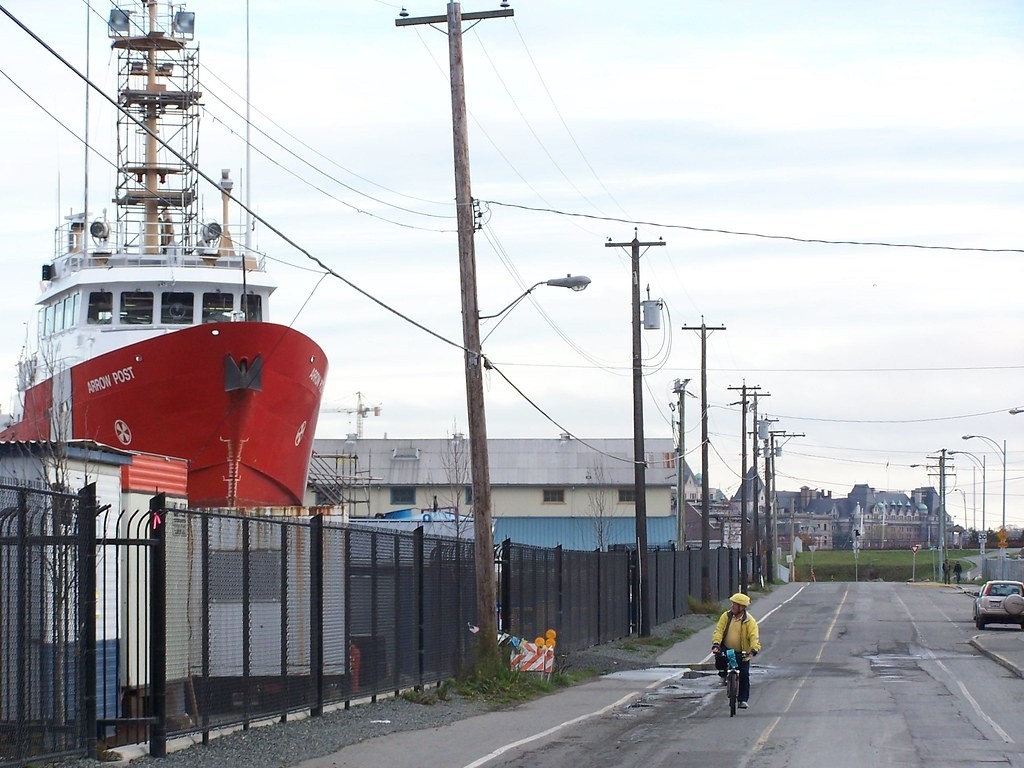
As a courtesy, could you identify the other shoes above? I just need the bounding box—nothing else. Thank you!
[719,675,728,686]
[738,701,748,708]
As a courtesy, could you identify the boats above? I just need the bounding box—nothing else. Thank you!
[0,0,333,512]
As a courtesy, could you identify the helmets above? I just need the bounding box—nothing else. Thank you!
[730,593,750,607]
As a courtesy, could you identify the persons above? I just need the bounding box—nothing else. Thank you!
[942,559,962,585]
[809,572,816,582]
[712,593,760,707]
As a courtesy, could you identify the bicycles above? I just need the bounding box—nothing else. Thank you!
[716,650,754,717]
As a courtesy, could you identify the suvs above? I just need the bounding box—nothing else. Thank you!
[973,579,1024,630]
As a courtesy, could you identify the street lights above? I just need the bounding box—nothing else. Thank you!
[955,488,968,533]
[948,450,988,580]
[962,435,1006,581]
[467,274,592,673]
